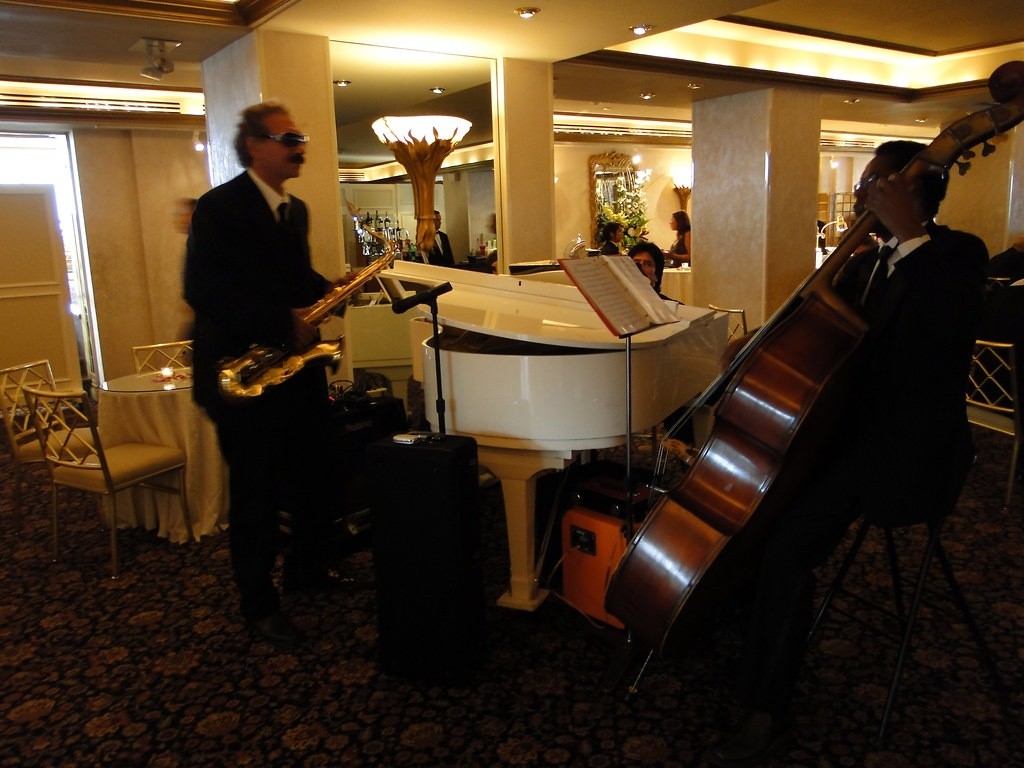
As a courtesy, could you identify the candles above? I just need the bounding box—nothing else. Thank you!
[160,366,173,378]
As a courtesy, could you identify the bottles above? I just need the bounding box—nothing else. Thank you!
[480,240,497,256]
[477,238,480,248]
[472,247,476,256]
[366,209,411,252]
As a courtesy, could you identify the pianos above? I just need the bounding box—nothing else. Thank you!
[376,253,732,611]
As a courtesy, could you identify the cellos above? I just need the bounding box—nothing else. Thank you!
[600,56,1024,706]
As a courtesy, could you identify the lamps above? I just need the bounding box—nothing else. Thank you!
[369,114,472,258]
[672,174,691,213]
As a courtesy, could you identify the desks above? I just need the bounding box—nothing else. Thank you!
[88,365,228,545]
[661,265,695,305]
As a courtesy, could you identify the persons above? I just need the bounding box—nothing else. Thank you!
[416,209,457,268]
[171,198,198,341]
[601,222,628,257]
[176,101,369,646]
[668,211,691,267]
[628,240,686,306]
[711,141,988,768]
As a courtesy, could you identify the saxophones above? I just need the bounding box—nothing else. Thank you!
[217,197,402,401]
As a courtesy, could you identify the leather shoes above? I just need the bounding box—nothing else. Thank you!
[239,602,304,648]
[280,572,334,597]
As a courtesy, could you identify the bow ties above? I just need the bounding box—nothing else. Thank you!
[436,231,439,235]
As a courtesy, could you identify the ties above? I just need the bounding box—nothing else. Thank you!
[276,202,292,256]
[857,245,897,315]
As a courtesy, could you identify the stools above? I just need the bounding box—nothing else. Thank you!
[803,438,1003,740]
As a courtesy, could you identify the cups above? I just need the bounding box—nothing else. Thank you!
[682,262,688,267]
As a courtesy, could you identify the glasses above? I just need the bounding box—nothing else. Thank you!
[263,130,307,148]
[853,175,879,191]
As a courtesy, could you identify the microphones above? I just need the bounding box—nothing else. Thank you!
[391,282,453,314]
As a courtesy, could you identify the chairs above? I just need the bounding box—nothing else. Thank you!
[132,339,195,374]
[0,358,108,537]
[706,303,749,342]
[18,383,202,583]
[964,340,1024,515]
